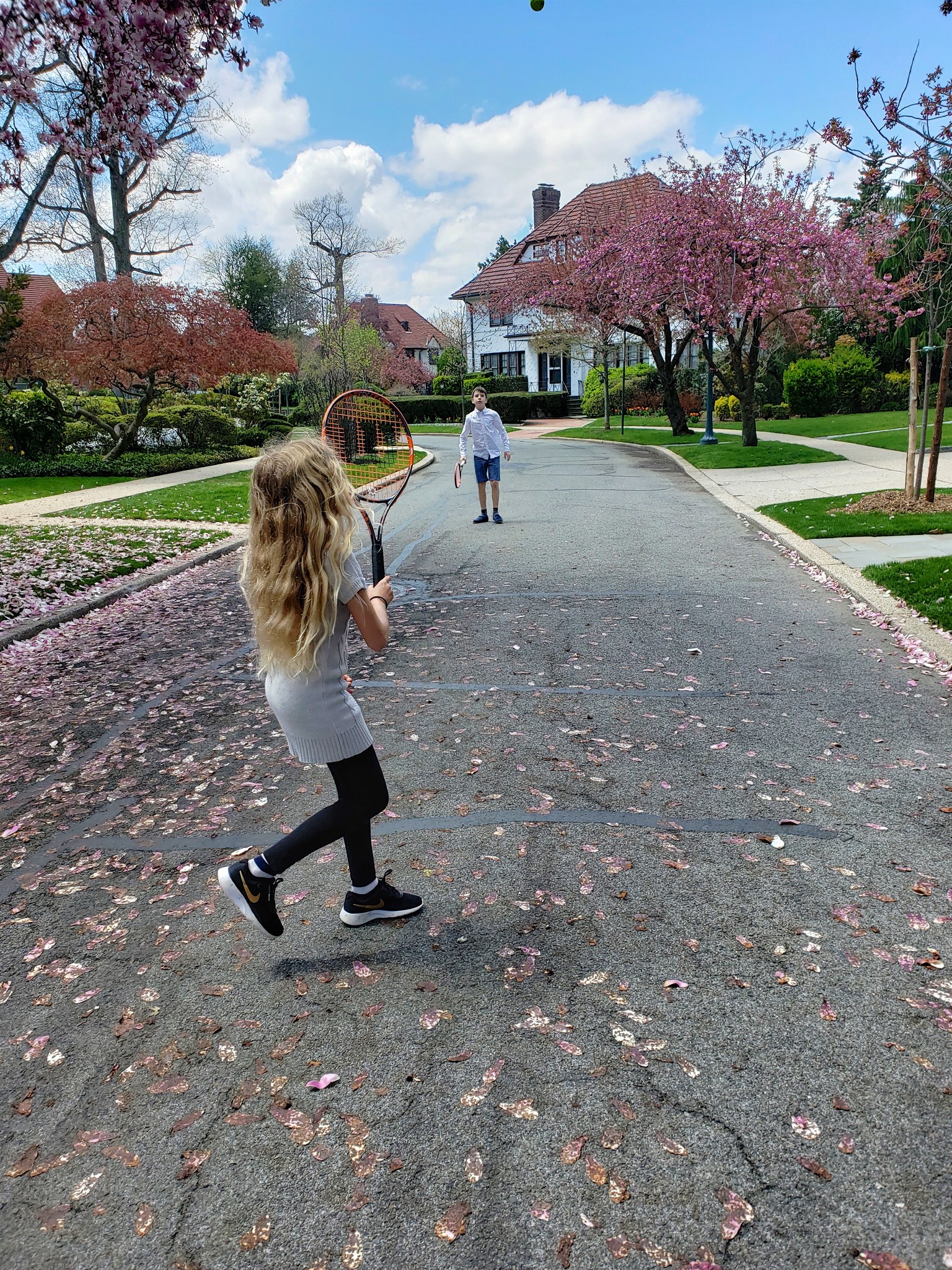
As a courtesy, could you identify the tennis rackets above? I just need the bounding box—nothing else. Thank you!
[454,458,466,489]
[320,387,415,591]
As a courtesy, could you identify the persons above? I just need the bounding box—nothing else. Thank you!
[458,386,511,523]
[217,439,425,937]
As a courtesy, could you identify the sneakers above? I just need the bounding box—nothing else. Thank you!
[339,869,424,926]
[218,858,284,938]
[473,513,489,524]
[493,511,503,523]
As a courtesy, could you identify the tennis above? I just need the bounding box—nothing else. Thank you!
[529,0,545,12]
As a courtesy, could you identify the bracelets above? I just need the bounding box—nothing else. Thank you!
[370,596,387,609]
